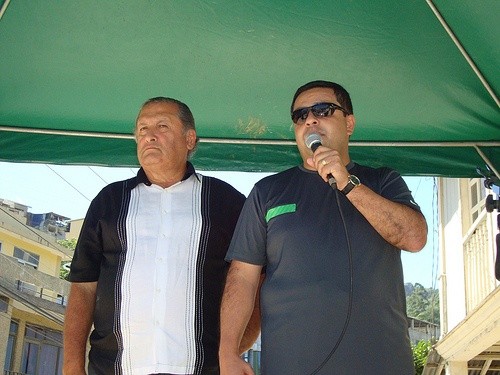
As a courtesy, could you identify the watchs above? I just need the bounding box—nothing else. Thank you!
[339,173,361,195]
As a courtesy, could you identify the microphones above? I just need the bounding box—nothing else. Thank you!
[305,132,337,191]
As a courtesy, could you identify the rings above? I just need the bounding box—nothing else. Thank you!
[321,158,328,166]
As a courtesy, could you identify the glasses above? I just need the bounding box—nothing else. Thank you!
[291,103,349,124]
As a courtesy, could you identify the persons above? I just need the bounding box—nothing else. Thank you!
[218,79,430,375]
[62,96,260,375]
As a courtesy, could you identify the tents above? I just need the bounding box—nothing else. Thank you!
[0,1,500,180]
